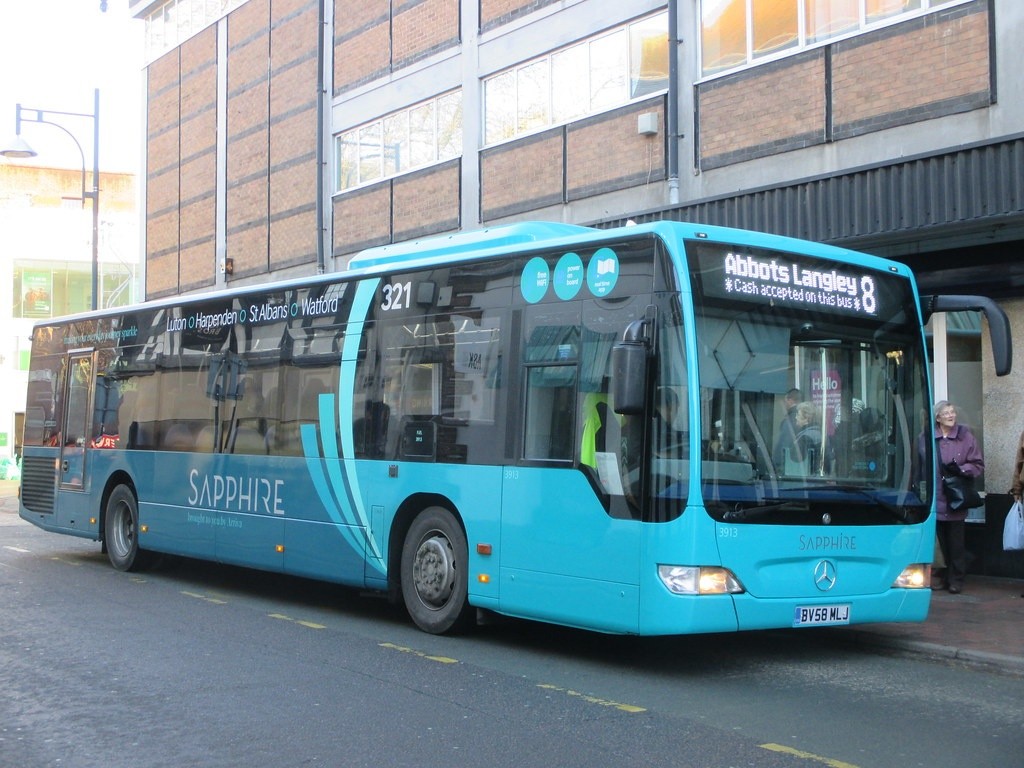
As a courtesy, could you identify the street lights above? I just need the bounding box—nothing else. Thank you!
[0,88,101,310]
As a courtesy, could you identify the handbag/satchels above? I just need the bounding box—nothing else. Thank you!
[935,439,984,511]
[1003,491,1024,551]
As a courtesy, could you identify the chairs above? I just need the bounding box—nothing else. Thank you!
[595,401,642,511]
[164,422,276,456]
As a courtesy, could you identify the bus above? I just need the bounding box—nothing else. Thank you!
[19,219,1013,638]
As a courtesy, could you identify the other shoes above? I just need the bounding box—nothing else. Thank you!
[949,584,961,593]
[931,578,947,590]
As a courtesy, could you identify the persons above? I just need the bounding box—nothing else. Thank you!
[241,377,328,435]
[1012,430,1024,597]
[932,400,985,592]
[917,409,930,501]
[630,387,721,520]
[772,388,888,479]
